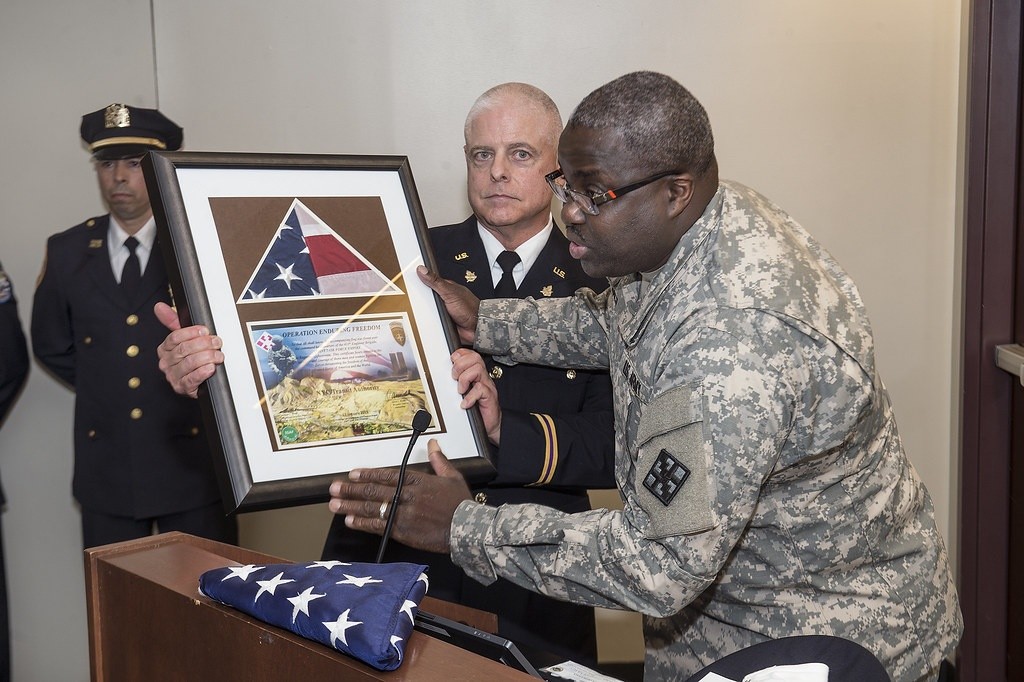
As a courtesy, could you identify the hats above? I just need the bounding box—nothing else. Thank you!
[81,102,183,160]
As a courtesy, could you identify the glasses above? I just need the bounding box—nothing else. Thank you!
[544,167,689,216]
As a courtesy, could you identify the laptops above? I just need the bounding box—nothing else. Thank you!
[415,610,625,682]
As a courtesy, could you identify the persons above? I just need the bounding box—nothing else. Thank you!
[154,81,617,669]
[327,70,964,682]
[268,335,297,383]
[30,102,240,552]
[0,259,31,682]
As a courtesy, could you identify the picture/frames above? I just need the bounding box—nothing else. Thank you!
[138,150,498,514]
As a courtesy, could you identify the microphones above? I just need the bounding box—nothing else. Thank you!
[376,409,433,565]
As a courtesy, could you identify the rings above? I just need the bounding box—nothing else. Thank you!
[378,501,389,518]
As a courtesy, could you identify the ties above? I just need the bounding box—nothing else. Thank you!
[120,236,141,284]
[493,251,523,297]
